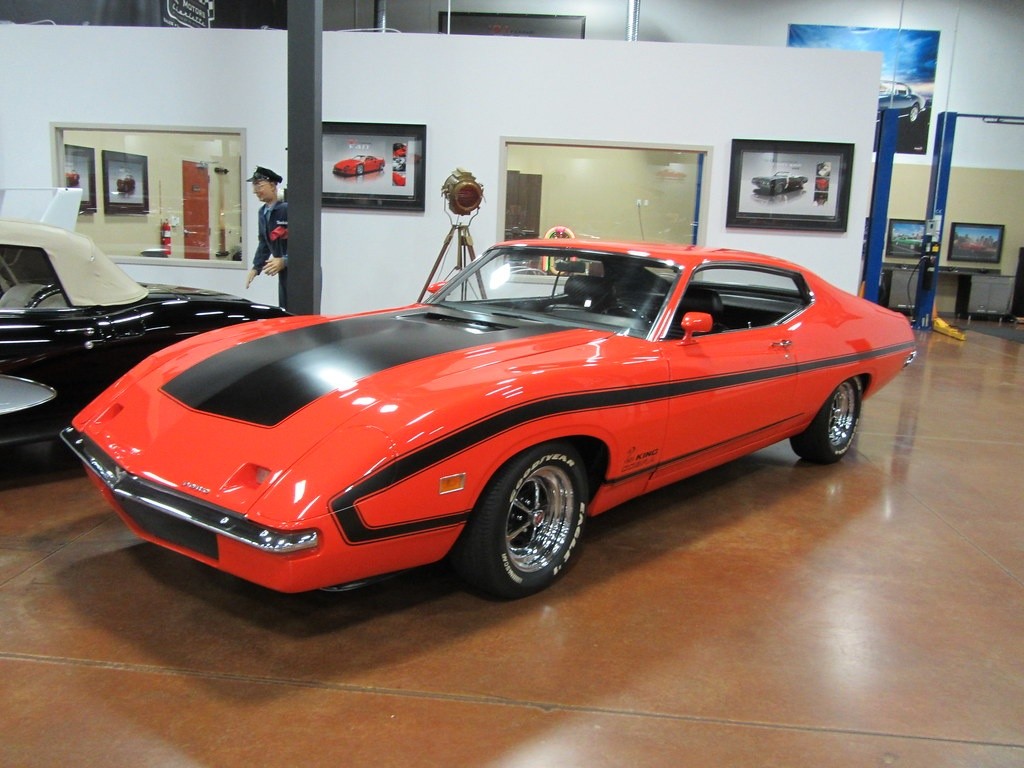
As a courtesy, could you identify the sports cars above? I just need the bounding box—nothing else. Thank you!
[333,154,386,176]
[59,235,917,604]
[1,218,300,449]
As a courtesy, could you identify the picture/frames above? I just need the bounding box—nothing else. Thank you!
[727,138,856,232]
[64,144,98,216]
[947,220,1005,264]
[885,218,925,260]
[322,121,427,212]
[101,149,150,215]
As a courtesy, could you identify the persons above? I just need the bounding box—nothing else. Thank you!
[244,165,288,309]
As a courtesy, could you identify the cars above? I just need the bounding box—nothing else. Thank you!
[118,175,135,192]
[954,239,987,255]
[893,234,923,249]
[751,170,808,194]
[876,80,927,124]
[65,169,80,186]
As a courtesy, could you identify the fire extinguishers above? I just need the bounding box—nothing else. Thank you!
[161,217,172,255]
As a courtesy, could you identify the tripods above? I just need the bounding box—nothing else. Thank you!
[416,225,488,303]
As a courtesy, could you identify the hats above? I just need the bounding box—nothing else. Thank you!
[246,165,283,185]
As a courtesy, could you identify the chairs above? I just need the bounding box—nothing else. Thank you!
[665,288,728,339]
[544,275,614,312]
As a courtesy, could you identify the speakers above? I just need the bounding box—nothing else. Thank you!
[956,275,1014,318]
[879,268,920,312]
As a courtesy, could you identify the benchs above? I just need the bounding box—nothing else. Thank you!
[1,283,68,308]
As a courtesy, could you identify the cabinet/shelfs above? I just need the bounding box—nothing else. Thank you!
[954,274,1016,323]
